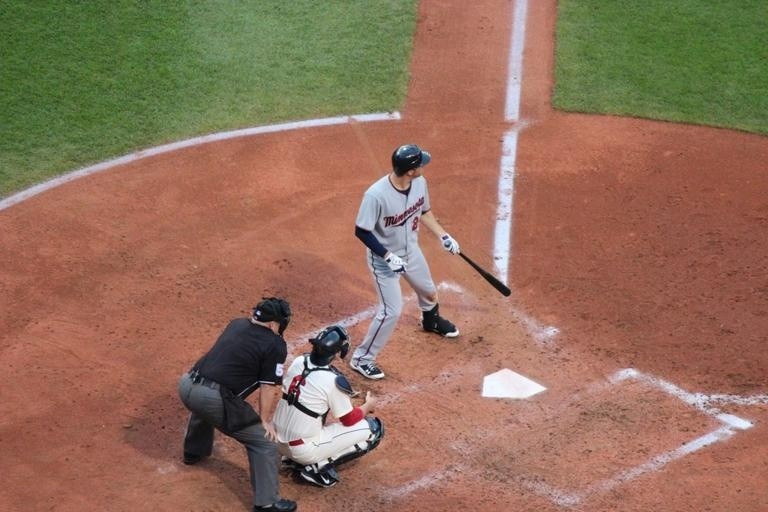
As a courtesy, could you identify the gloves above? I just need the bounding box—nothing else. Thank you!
[385,253,408,274]
[441,234,460,255]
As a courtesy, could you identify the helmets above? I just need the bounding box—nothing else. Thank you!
[253,297,290,336]
[309,326,351,366]
[391,144,431,176]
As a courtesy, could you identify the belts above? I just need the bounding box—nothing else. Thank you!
[189,370,220,391]
[289,439,304,446]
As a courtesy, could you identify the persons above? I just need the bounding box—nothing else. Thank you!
[178,296,298,512]
[270,324,385,489]
[348,144,461,380]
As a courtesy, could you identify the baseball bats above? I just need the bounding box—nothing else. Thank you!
[444,240,511,299]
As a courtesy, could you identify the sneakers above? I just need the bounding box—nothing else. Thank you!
[423,317,459,338]
[252,455,337,512]
[350,358,384,379]
